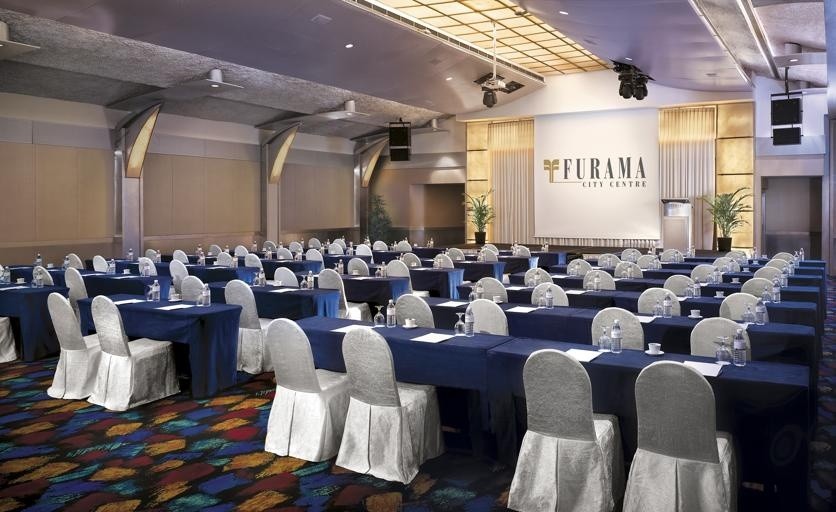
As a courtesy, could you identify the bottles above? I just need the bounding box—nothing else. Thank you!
[65,256,71,268]
[772,281,781,303]
[628,264,632,279]
[694,277,701,297]
[575,260,581,278]
[753,247,758,260]
[663,293,672,319]
[200,252,205,266]
[341,235,346,243]
[594,272,601,292]
[691,245,695,257]
[534,269,541,286]
[654,257,658,269]
[733,329,748,367]
[393,240,398,252]
[674,250,678,262]
[545,285,553,308]
[301,239,304,246]
[755,298,766,325]
[652,244,655,255]
[156,250,161,263]
[380,261,387,277]
[233,253,238,268]
[445,248,449,254]
[307,270,314,289]
[729,257,735,273]
[513,241,518,256]
[610,319,622,354]
[788,259,795,276]
[400,253,404,261]
[279,242,283,249]
[338,259,344,274]
[143,261,150,277]
[780,268,787,287]
[36,254,42,266]
[129,249,133,261]
[465,306,474,338]
[268,247,272,260]
[794,252,799,269]
[742,253,748,265]
[438,254,443,268]
[800,248,805,261]
[480,250,486,262]
[632,250,637,263]
[259,268,265,287]
[224,245,229,253]
[203,284,210,307]
[545,242,548,252]
[476,281,484,300]
[35,269,43,289]
[349,242,354,256]
[152,279,161,302]
[320,243,325,256]
[714,266,720,285]
[387,299,396,328]
[3,266,10,283]
[253,241,257,252]
[298,249,302,260]
[108,258,115,275]
[608,255,612,267]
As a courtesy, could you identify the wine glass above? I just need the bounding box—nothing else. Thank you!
[794,251,799,257]
[706,272,713,284]
[395,256,400,260]
[621,266,628,279]
[722,261,729,274]
[716,336,732,365]
[598,325,611,353]
[782,265,788,274]
[374,306,385,327]
[527,273,534,288]
[788,257,793,264]
[760,286,771,303]
[146,285,153,301]
[374,265,381,278]
[196,287,204,307]
[253,272,260,286]
[586,276,594,292]
[468,286,475,302]
[454,313,466,336]
[300,275,308,290]
[433,258,439,269]
[684,282,693,299]
[742,302,755,324]
[569,264,576,276]
[333,263,339,273]
[648,260,654,270]
[31,272,38,288]
[538,291,546,308]
[772,274,780,287]
[652,298,663,318]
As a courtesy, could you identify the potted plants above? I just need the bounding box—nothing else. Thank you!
[462,188,499,248]
[695,182,750,256]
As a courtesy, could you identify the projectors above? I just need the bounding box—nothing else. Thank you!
[481,77,506,90]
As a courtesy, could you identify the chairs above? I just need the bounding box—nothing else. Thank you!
[432,253,452,267]
[306,251,320,259]
[620,249,638,261]
[244,253,258,269]
[387,294,443,328]
[724,250,748,264]
[277,248,289,262]
[332,238,349,248]
[523,267,555,286]
[217,254,236,267]
[463,300,510,337]
[182,276,207,314]
[614,261,640,278]
[736,279,775,300]
[472,277,510,303]
[636,288,677,316]
[288,240,297,251]
[173,250,186,264]
[513,246,530,258]
[664,274,695,296]
[638,255,662,268]
[448,247,461,258]
[233,245,243,259]
[772,251,796,264]
[137,258,155,278]
[0,312,13,367]
[169,260,192,298]
[374,240,388,250]
[532,283,565,307]
[63,267,91,301]
[207,243,222,258]
[396,240,413,252]
[753,267,781,286]
[328,243,343,254]
[663,250,685,263]
[335,324,449,484]
[401,252,422,266]
[689,262,719,282]
[355,243,372,256]
[624,359,740,512]
[264,240,279,252]
[86,293,194,413]
[63,254,84,271]
[91,254,106,273]
[597,253,619,267]
[146,247,155,260]
[592,306,644,353]
[584,270,615,291]
[307,238,319,249]
[763,258,790,271]
[318,268,374,324]
[568,258,591,277]
[690,318,755,362]
[484,241,499,255]
[223,279,279,377]
[720,293,763,320]
[265,317,351,466]
[33,264,57,290]
[508,347,624,512]
[348,258,372,278]
[479,249,493,262]
[46,289,107,398]
[275,267,302,287]
[711,257,739,270]
[386,260,431,299]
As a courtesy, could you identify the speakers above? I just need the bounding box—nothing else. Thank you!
[770,91,803,146]
[389,120,412,161]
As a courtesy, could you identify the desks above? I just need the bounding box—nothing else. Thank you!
[551,303,746,358]
[700,263,821,285]
[411,290,546,338]
[377,244,449,256]
[607,287,734,318]
[377,266,464,301]
[509,251,568,264]
[426,259,505,286]
[243,249,300,255]
[148,262,230,278]
[474,254,539,274]
[513,263,649,289]
[294,312,507,466]
[2,283,70,363]
[259,259,323,275]
[90,257,136,274]
[296,272,411,303]
[487,334,750,485]
[14,263,76,286]
[169,254,260,267]
[583,265,693,282]
[739,254,826,264]
[622,256,717,268]
[621,271,748,295]
[202,284,341,321]
[304,254,374,265]
[79,290,241,400]
[80,272,152,296]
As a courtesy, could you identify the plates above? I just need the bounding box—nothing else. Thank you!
[492,301,504,304]
[121,273,132,275]
[714,296,727,299]
[688,315,703,319]
[742,271,751,273]
[730,282,741,284]
[212,264,220,266]
[262,250,268,251]
[761,258,769,260]
[402,325,418,329]
[168,299,183,303]
[409,265,420,268]
[350,274,361,276]
[45,266,55,269]
[644,350,665,355]
[13,282,27,285]
[752,264,759,266]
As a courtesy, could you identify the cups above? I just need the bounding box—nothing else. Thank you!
[47,263,53,267]
[171,294,179,301]
[405,318,415,327]
[353,270,359,275]
[410,262,417,266]
[208,252,212,255]
[716,291,724,296]
[263,248,266,251]
[753,260,758,264]
[744,268,749,271]
[762,255,767,258]
[124,269,129,274]
[690,310,700,317]
[493,295,500,302]
[16,278,24,283]
[732,278,739,282]
[213,261,218,265]
[648,343,661,353]
[273,280,281,286]
